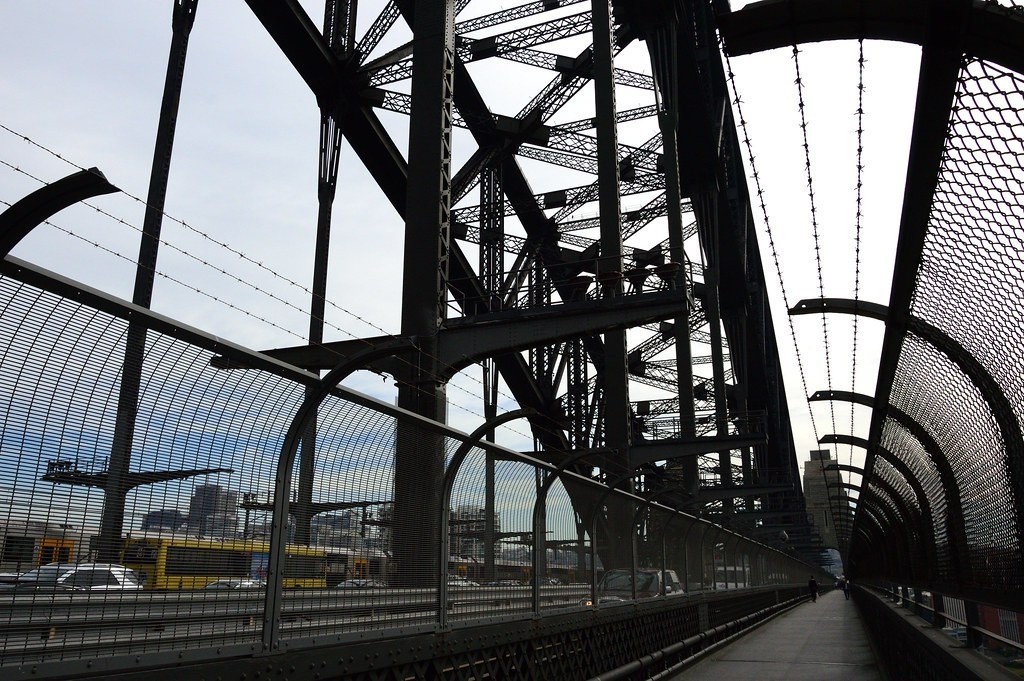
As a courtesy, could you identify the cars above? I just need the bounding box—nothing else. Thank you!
[334,579,387,588]
[0,571,24,589]
[447,573,563,586]
[202,578,268,590]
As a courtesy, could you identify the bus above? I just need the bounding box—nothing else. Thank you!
[116,537,327,592]
[768,573,788,585]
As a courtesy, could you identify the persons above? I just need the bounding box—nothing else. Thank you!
[843,581,849,600]
[809,576,818,603]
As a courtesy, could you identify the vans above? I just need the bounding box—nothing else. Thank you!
[710,566,751,590]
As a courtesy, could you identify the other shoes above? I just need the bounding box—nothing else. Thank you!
[846,598,849,600]
[813,599,816,603]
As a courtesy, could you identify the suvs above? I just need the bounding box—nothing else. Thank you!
[578,568,685,606]
[7,562,144,591]
